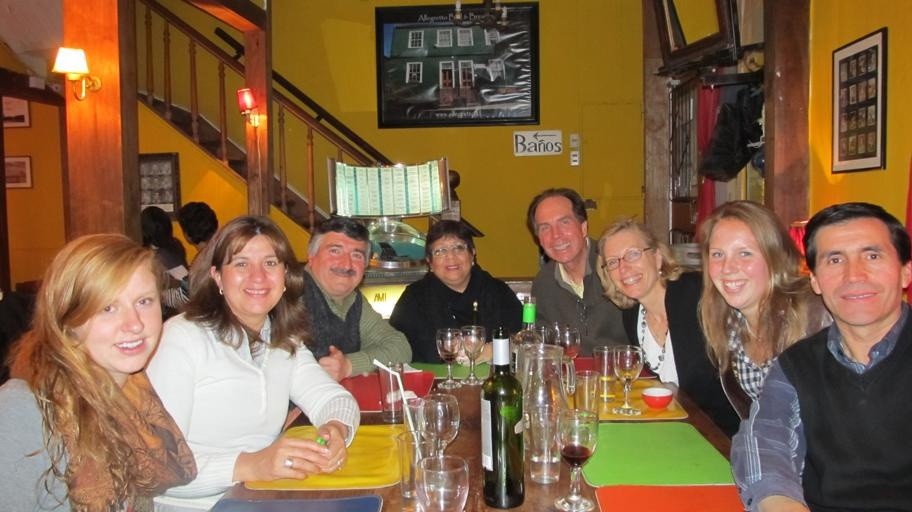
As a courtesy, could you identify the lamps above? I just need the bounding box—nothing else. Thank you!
[50,45,101,102]
[235,83,262,129]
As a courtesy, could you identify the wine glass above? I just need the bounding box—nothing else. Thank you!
[417,393,462,456]
[552,409,601,511]
[610,344,645,417]
[413,457,471,511]
[436,328,462,390]
[550,321,583,367]
[459,324,486,385]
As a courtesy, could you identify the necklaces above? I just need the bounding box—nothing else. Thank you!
[641,309,669,371]
[745,321,764,342]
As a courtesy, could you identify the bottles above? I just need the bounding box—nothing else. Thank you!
[480,327,526,510]
[514,296,542,375]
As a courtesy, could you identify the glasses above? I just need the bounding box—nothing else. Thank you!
[601,246,655,271]
[431,242,468,257]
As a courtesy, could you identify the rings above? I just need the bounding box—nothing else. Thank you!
[284,458,292,468]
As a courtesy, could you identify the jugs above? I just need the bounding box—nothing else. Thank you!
[525,345,577,451]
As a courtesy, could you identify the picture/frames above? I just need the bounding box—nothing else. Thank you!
[374,0,542,129]
[830,24,889,175]
[137,152,181,220]
[4,153,34,190]
[0,96,33,129]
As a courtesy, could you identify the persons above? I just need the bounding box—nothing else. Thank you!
[388,219,523,366]
[596,218,739,441]
[696,199,834,422]
[729,202,911,512]
[281,217,413,432]
[0,233,199,512]
[141,206,187,279]
[159,201,219,309]
[143,214,361,512]
[528,187,631,357]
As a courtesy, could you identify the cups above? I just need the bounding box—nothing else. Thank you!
[377,362,404,422]
[641,389,673,408]
[591,347,616,403]
[575,370,599,414]
[395,431,430,499]
[527,405,561,487]
